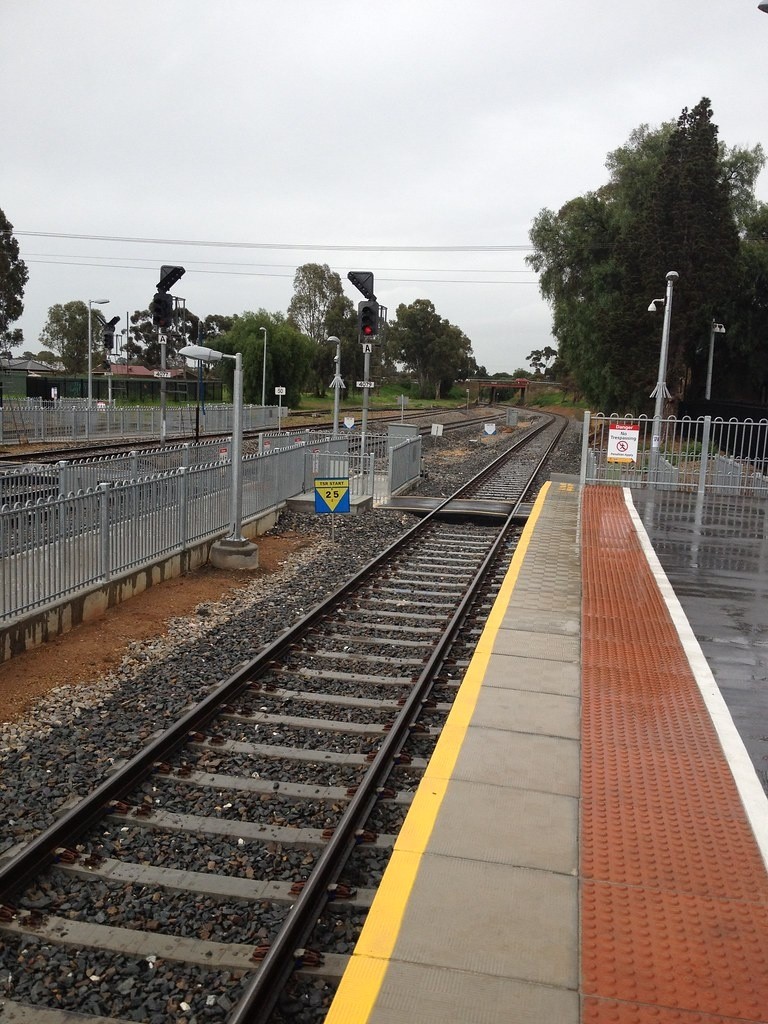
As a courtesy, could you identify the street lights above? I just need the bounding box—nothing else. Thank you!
[177,346,248,541]
[706,317,726,401]
[87,299,108,401]
[259,327,269,406]
[328,335,342,438]
[649,270,680,489]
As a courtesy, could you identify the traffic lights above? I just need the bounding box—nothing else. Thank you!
[152,296,171,327]
[104,331,113,348]
[361,305,374,336]
